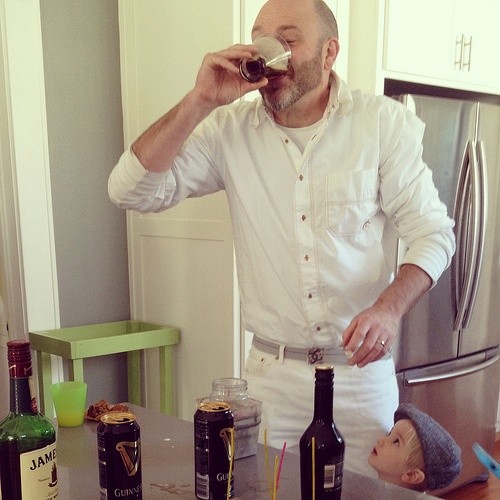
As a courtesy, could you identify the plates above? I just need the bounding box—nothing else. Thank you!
[85,409,99,421]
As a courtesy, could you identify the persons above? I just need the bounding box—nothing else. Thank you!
[108,0,458,481]
[366,404,463,494]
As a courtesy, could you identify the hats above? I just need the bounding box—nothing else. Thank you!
[393,404,462,490]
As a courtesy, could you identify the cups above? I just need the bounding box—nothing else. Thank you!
[239,33,292,84]
[50,382,87,426]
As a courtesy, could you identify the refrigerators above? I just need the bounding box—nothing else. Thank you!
[390,95,500,495]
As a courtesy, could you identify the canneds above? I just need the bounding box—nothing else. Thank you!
[96,412,142,500]
[194,402,235,500]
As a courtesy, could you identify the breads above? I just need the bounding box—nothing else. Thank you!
[87,399,129,418]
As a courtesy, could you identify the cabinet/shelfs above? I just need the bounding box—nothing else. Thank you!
[380,0,500,97]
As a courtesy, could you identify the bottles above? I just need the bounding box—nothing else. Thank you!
[0,339,58,500]
[299,363,345,500]
[208,378,251,409]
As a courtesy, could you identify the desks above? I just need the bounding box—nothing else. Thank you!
[43,402,446,500]
[26,318,183,415]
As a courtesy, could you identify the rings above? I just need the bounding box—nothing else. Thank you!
[377,340,385,347]
